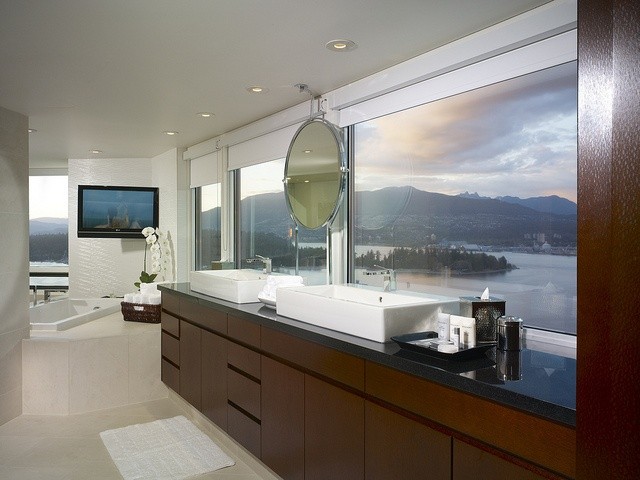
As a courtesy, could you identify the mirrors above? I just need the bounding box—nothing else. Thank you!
[284,119,345,226]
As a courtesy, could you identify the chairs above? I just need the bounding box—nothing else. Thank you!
[227,315,261,473]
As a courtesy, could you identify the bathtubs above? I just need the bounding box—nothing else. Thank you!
[29,299,124,331]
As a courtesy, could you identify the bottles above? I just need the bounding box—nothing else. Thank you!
[495,348,519,381]
[495,315,524,351]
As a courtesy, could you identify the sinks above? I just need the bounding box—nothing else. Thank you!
[275,284,460,343]
[190,268,303,304]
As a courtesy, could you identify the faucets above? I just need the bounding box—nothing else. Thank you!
[43,288,68,303]
[363,264,397,291]
[245,254,273,274]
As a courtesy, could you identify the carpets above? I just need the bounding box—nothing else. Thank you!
[101,416,235,480]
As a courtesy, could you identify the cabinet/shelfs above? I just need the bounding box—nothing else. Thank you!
[161,291,180,397]
[261,327,365,480]
[365,361,577,480]
[180,296,228,445]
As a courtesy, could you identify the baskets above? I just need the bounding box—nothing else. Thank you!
[121,301,161,324]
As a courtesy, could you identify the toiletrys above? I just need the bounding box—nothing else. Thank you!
[438,313,450,340]
[454,328,460,352]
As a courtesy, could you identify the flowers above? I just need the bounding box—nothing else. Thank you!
[135,227,171,291]
